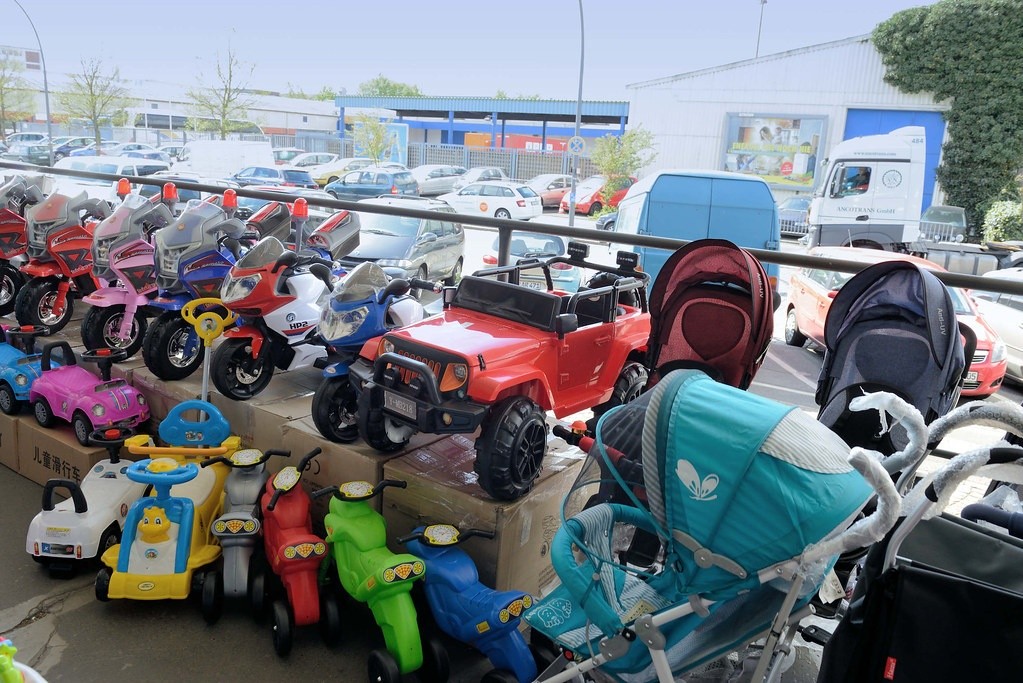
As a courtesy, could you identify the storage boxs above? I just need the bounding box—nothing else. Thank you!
[0,300,601,660]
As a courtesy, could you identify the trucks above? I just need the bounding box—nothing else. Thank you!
[797,124,1022,279]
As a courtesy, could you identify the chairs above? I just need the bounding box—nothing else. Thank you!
[544,242,558,253]
[362,174,372,183]
[180,189,196,202]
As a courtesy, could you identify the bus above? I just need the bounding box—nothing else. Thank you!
[112,108,273,150]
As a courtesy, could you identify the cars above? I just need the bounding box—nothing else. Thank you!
[560,173,639,217]
[596,212,619,233]
[522,173,582,207]
[480,227,584,295]
[453,166,510,194]
[918,205,976,244]
[434,178,544,222]
[281,153,339,178]
[960,273,1023,390]
[777,194,812,237]
[411,163,467,197]
[322,165,420,203]
[307,156,375,189]
[782,245,1008,401]
[272,147,306,165]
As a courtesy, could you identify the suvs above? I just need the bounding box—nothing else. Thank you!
[323,193,466,303]
[229,184,340,232]
[347,235,651,504]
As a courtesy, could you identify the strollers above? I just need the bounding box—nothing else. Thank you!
[550,232,782,580]
[516,364,928,683]
[818,398,1023,683]
[787,255,979,622]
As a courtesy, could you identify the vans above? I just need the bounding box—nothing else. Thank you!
[609,168,783,314]
[173,138,277,174]
[44,154,170,207]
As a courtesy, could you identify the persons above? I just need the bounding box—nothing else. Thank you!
[844,168,871,191]
[744,126,815,177]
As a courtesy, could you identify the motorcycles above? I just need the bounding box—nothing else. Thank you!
[1,131,442,445]
